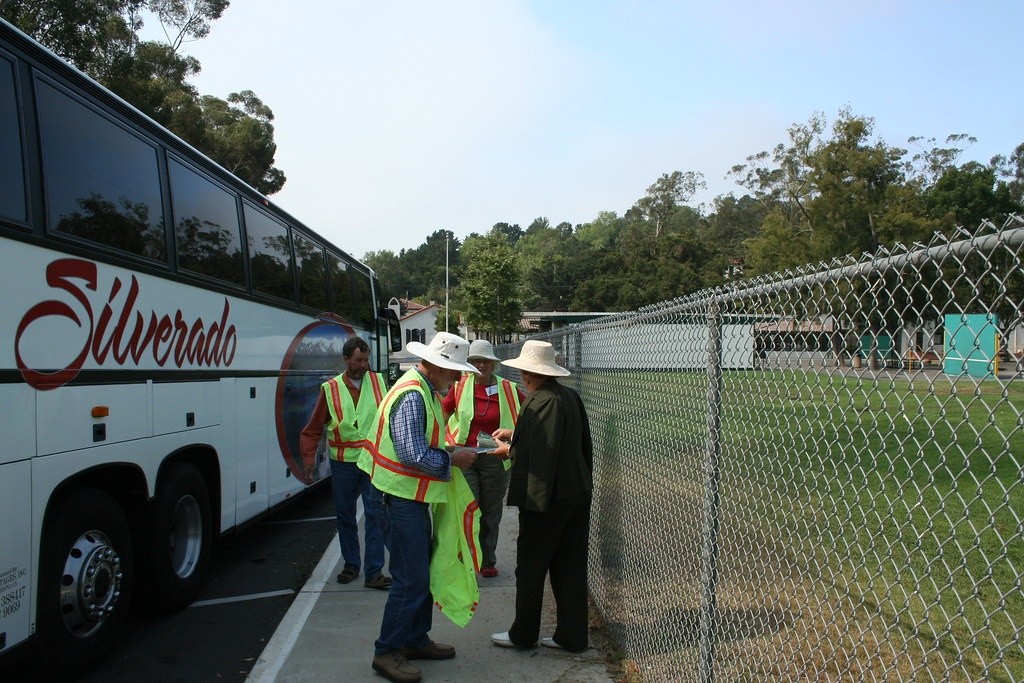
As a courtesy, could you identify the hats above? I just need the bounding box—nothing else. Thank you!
[501,340,572,376]
[466,340,501,362]
[406,331,482,375]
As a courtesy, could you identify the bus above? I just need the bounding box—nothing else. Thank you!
[0,18,402,682]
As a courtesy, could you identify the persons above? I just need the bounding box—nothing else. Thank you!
[357,332,477,683]
[299,337,392,587]
[442,340,526,577]
[492,340,592,653]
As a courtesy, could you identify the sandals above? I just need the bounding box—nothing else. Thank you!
[365,574,392,588]
[337,567,359,584]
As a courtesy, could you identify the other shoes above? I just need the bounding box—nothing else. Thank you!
[480,566,498,577]
[492,631,539,648]
[372,650,421,683]
[405,638,455,659]
[541,637,565,648]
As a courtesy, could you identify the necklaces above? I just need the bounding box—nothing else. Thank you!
[475,378,491,416]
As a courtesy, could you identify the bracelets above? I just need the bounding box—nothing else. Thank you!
[507,446,511,458]
